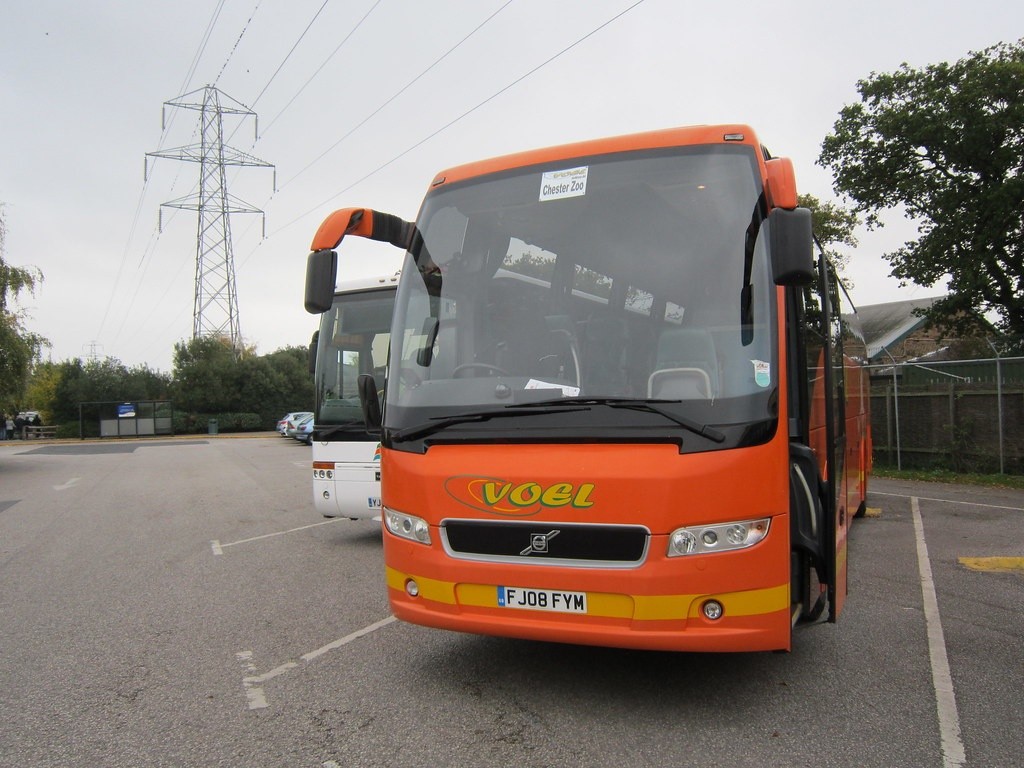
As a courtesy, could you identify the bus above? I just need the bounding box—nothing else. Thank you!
[301,121,875,656]
[307,260,684,523]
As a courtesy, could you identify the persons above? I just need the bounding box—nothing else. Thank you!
[14,416,24,440]
[23,417,31,440]
[32,415,42,438]
[5,417,16,440]
[0,415,7,440]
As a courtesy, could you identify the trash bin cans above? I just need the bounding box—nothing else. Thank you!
[209,418,219,434]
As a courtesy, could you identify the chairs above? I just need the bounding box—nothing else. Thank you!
[547,312,763,400]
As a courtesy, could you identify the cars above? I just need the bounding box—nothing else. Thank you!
[275,411,314,441]
[296,414,315,446]
